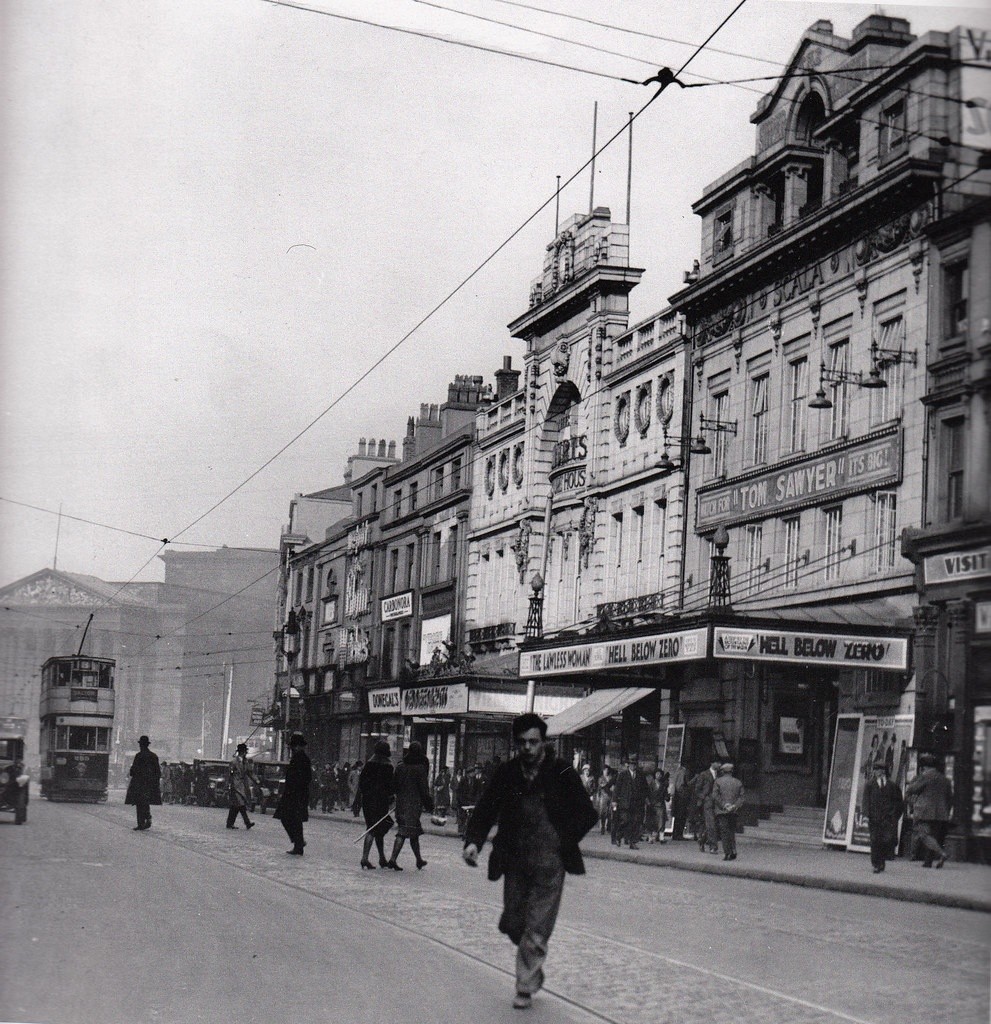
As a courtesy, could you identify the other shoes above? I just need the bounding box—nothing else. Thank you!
[145,819,152,828]
[512,993,532,1008]
[698,839,705,852]
[287,846,303,855]
[133,825,144,830]
[227,824,239,829]
[730,853,737,860]
[389,861,404,871]
[247,822,255,829]
[874,863,885,873]
[417,860,427,870]
[923,863,933,867]
[630,844,639,850]
[723,852,730,861]
[710,849,718,854]
[936,853,949,868]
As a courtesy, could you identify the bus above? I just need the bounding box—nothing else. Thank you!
[39,612,115,804]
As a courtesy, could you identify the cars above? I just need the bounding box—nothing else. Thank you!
[245,757,288,814]
[193,759,231,806]
[0,717,30,826]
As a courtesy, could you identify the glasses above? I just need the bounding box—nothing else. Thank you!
[518,737,538,746]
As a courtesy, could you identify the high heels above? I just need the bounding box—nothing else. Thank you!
[360,860,376,869]
[379,860,388,869]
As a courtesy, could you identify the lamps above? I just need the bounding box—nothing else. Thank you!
[653,428,699,468]
[807,361,863,408]
[690,410,738,454]
[860,340,917,388]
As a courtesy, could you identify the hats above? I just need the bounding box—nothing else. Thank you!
[294,734,307,746]
[628,755,639,766]
[138,736,151,745]
[236,744,249,751]
[721,763,734,773]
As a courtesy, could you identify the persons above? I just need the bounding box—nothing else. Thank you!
[273,731,312,856]
[224,743,260,829]
[860,731,907,785]
[713,763,746,861]
[60,672,66,686]
[463,712,599,1008]
[580,758,722,854]
[125,736,163,830]
[387,749,436,871]
[309,760,364,817]
[410,740,504,832]
[907,756,952,868]
[160,761,209,806]
[862,760,904,872]
[359,741,396,869]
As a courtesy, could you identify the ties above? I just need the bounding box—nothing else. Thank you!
[880,778,884,789]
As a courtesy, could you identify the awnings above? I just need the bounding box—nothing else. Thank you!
[542,688,656,736]
